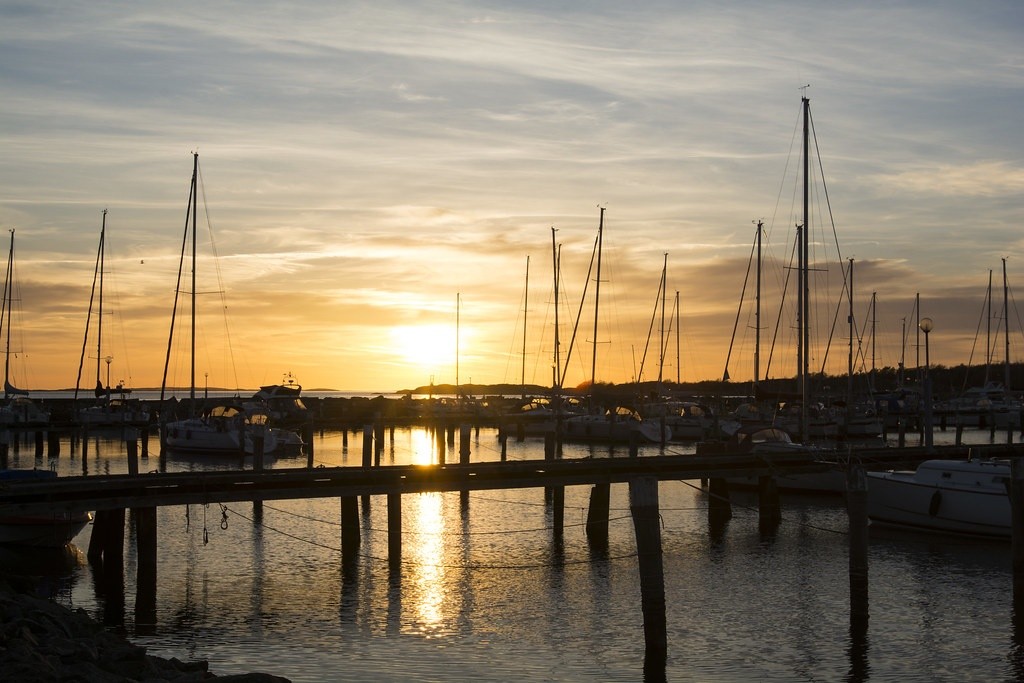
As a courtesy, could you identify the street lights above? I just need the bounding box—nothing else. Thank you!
[920,317,934,384]
[106,356,113,412]
[204,371,208,399]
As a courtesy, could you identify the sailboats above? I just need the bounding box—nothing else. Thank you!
[0,209,158,425]
[498,84,1023,446]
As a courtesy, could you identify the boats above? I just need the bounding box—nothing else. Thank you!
[0,438,93,549]
[164,386,308,457]
[720,461,870,497]
[844,448,1023,545]
[696,390,792,456]
